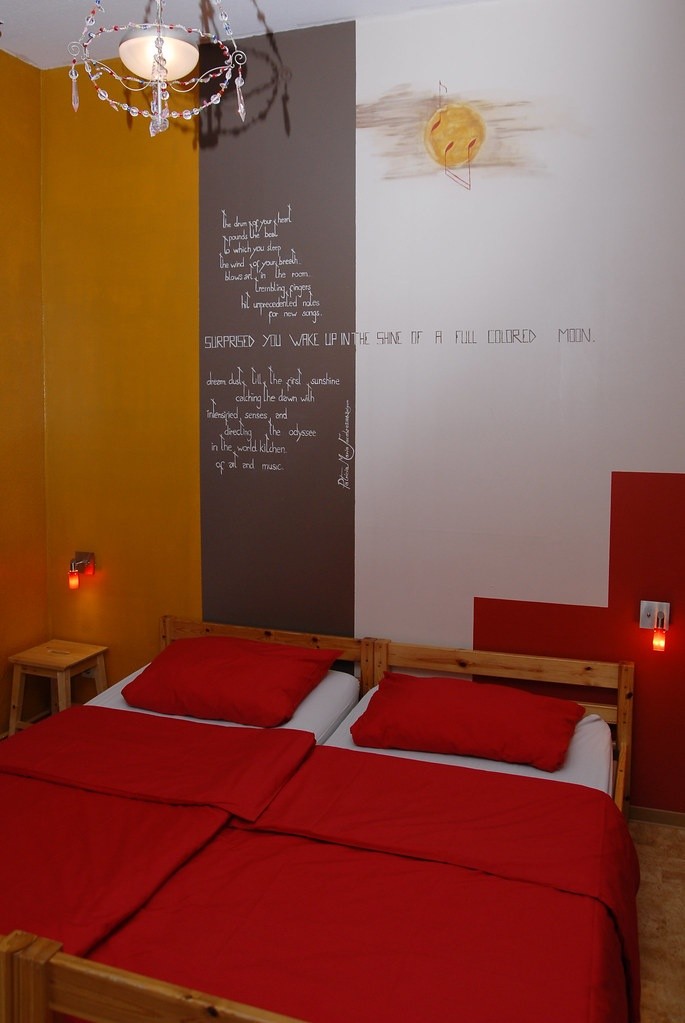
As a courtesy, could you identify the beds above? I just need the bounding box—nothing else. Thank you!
[12,639,641,1023]
[0,615,377,1023]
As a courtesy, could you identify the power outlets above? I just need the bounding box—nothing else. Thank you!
[640,600,670,632]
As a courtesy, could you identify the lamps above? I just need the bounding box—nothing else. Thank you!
[68,552,94,591]
[653,613,665,652]
[69,0,247,138]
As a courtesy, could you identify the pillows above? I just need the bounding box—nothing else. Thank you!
[120,636,348,728]
[350,671,586,774]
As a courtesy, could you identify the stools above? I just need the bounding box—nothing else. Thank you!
[7,638,108,737]
